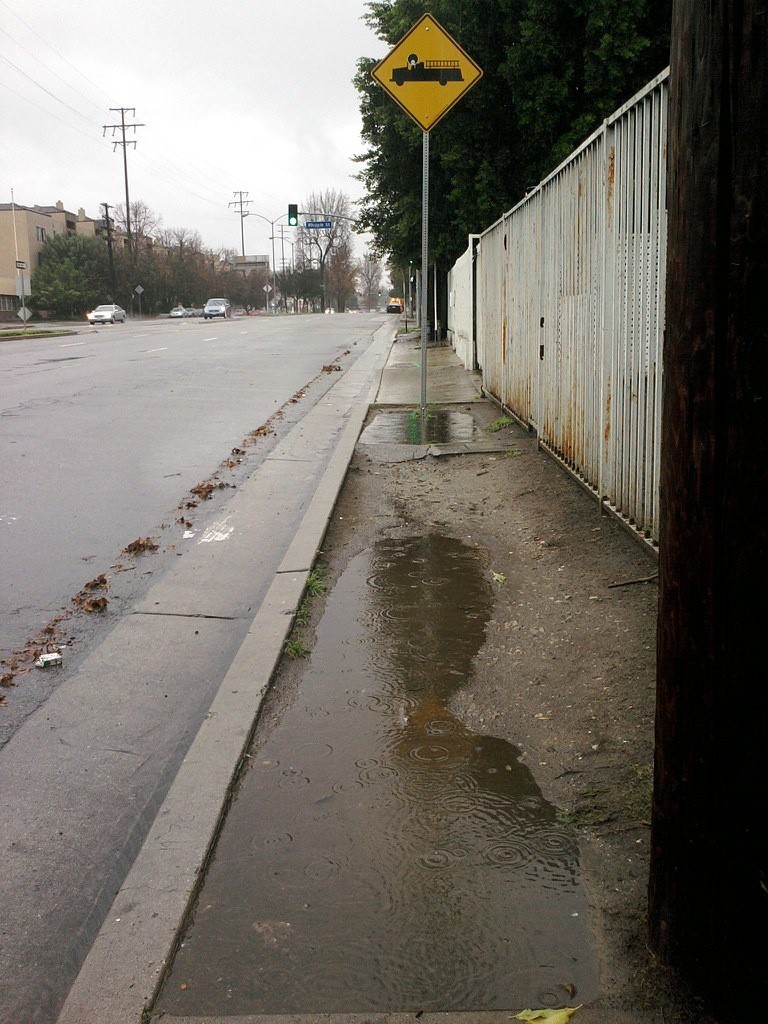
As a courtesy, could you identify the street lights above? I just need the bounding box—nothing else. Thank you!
[241,212,309,316]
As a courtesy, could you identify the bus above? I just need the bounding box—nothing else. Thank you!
[389,297,402,304]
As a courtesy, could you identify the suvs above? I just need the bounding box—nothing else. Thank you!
[203,298,231,319]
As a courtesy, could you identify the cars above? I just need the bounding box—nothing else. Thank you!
[324,308,335,314]
[187,308,206,317]
[88,305,127,324]
[350,308,376,313]
[387,303,403,313]
[169,307,184,317]
[234,308,247,317]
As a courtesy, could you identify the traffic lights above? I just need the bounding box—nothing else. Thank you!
[290,204,298,227]
[378,291,382,296]
